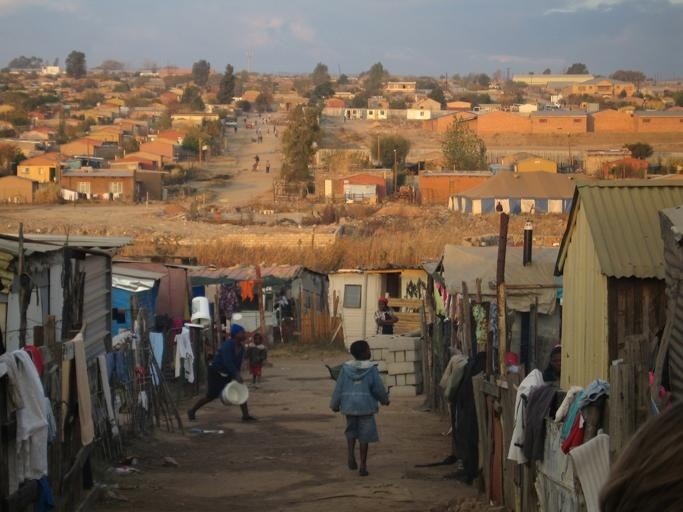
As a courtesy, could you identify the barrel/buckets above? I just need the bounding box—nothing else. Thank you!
[190,296,211,327]
[220,379,250,407]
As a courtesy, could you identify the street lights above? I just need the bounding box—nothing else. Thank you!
[392,149,398,193]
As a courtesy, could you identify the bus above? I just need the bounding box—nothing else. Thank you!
[224,115,237,127]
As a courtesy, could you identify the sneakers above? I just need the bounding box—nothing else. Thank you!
[241,417,256,421]
[187,409,195,420]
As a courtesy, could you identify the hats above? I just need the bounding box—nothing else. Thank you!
[378,297,388,305]
[231,324,243,336]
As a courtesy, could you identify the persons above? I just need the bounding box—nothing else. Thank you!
[243,333,268,382]
[439,337,468,442]
[187,323,261,421]
[595,399,683,511]
[328,340,389,476]
[373,297,398,335]
[541,344,561,384]
